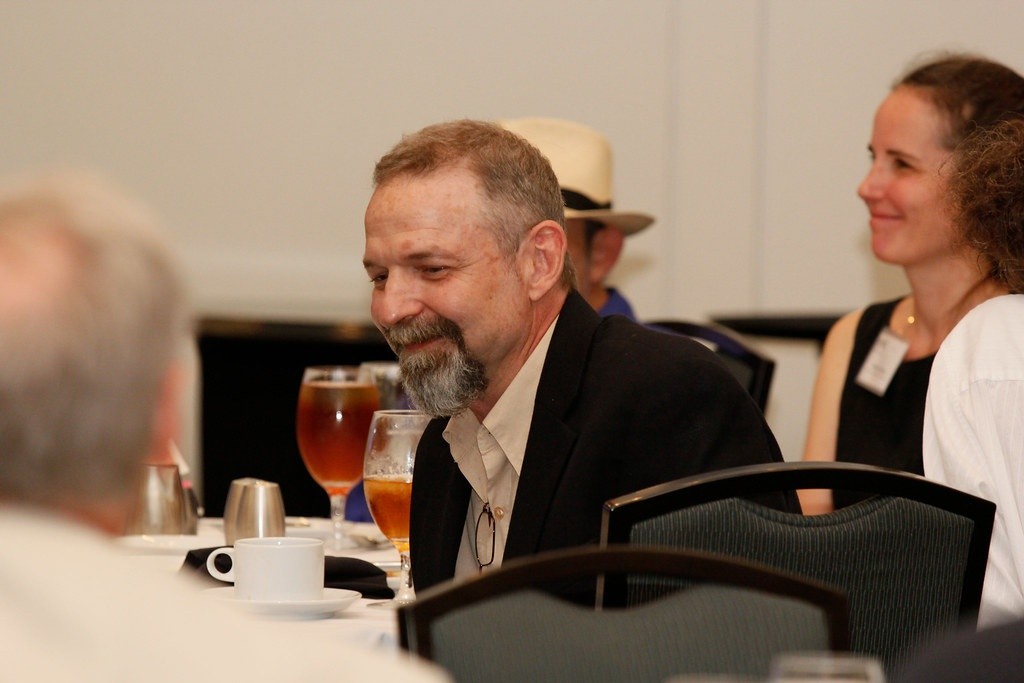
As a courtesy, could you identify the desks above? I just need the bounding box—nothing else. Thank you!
[128,516,415,611]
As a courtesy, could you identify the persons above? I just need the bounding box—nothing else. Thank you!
[795,57,1024,630]
[362,117,802,610]
[0,178,455,683]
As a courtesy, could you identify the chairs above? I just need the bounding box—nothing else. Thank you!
[397,320,997,683]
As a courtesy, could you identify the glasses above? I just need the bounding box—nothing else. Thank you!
[475,502,495,574]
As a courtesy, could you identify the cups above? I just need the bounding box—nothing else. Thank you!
[182,488,198,535]
[224,478,286,545]
[140,463,184,534]
[207,536,325,602]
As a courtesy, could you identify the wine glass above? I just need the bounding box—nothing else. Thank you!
[297,365,379,557]
[362,410,433,608]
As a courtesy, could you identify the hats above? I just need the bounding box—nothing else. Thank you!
[498,119,653,236]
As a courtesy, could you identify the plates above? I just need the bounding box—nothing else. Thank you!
[201,586,363,618]
[115,534,224,555]
